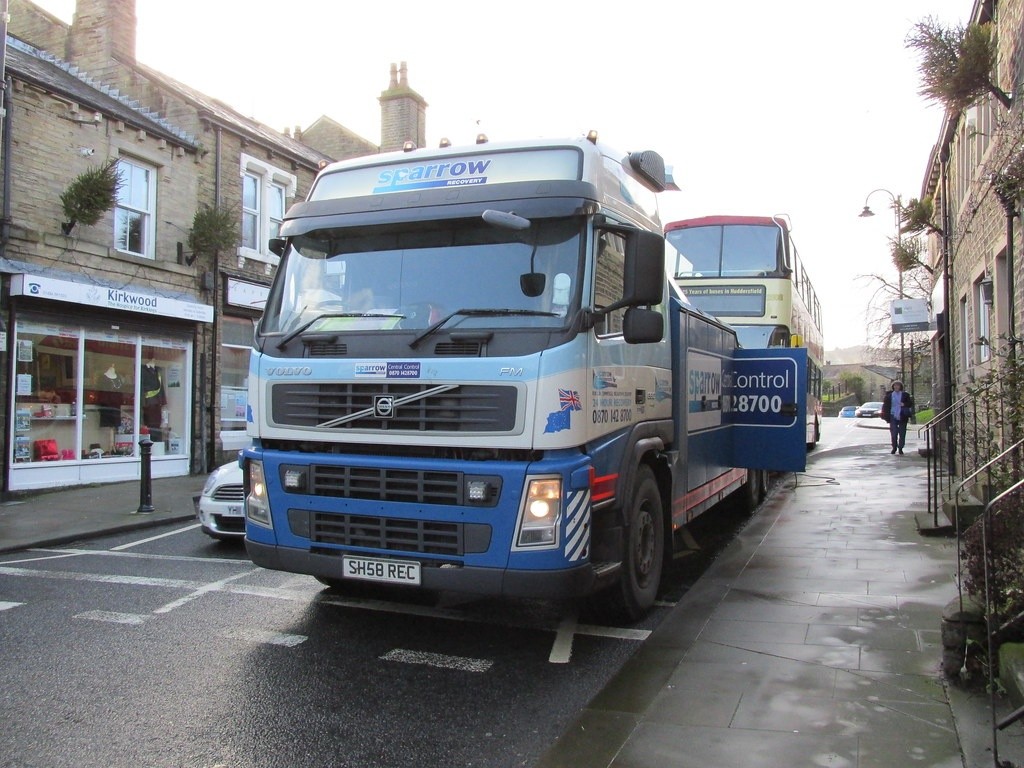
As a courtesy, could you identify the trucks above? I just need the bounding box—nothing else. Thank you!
[234,130,810,626]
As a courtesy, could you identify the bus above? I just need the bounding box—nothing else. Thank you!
[664,210,825,452]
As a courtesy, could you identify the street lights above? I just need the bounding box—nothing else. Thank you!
[857,188,905,392]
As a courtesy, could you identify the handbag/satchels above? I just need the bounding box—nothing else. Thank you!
[901,408,912,418]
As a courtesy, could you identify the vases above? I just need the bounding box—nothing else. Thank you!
[125,428,131,434]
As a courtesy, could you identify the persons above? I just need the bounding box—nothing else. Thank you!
[141,358,168,442]
[881,380,912,454]
[94,367,127,453]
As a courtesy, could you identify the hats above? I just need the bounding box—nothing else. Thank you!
[892,380,904,388]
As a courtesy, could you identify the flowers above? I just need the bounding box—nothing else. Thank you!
[117,417,133,433]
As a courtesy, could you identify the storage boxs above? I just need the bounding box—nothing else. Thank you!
[150,442,165,456]
[164,438,181,453]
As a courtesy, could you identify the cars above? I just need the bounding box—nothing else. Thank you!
[838,405,861,418]
[854,401,884,418]
[195,461,245,541]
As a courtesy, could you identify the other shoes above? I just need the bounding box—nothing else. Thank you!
[891,447,897,454]
[899,447,904,455]
